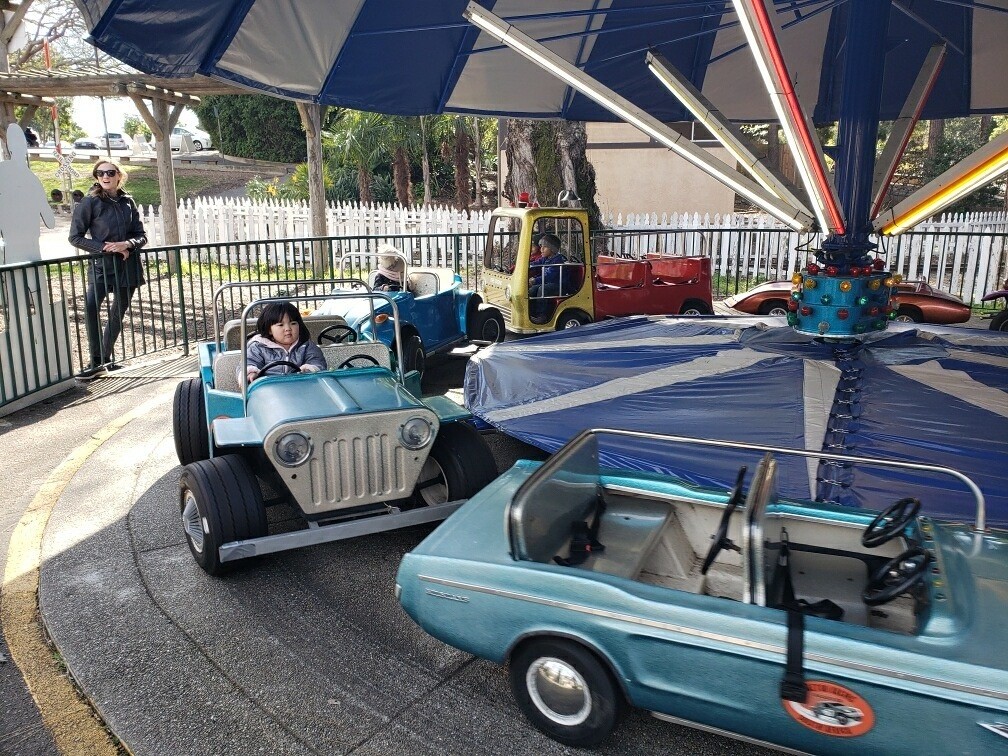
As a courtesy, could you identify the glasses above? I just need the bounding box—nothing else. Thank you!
[96,170,118,177]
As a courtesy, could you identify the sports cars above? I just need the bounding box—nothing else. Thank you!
[724,276,973,325]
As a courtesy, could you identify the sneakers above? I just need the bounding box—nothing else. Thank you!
[87,356,125,370]
[74,364,108,379]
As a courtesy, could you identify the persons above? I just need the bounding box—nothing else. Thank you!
[372,245,413,293]
[68,159,148,380]
[507,232,569,323]
[237,301,328,388]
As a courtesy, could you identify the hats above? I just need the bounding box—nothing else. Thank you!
[531,232,545,249]
[539,234,562,255]
[378,244,406,273]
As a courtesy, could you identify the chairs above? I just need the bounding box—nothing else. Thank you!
[916,276,926,292]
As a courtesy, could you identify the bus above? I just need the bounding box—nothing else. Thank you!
[479,204,715,335]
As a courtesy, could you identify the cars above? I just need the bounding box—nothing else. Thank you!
[151,127,217,151]
[74,138,101,149]
[171,278,500,578]
[393,426,1008,756]
[304,252,507,390]
[100,132,133,150]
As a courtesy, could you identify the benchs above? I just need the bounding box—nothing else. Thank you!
[529,261,587,303]
[368,267,455,300]
[216,342,394,393]
[646,253,707,286]
[505,433,695,576]
[742,449,869,629]
[223,314,351,352]
[597,255,650,292]
